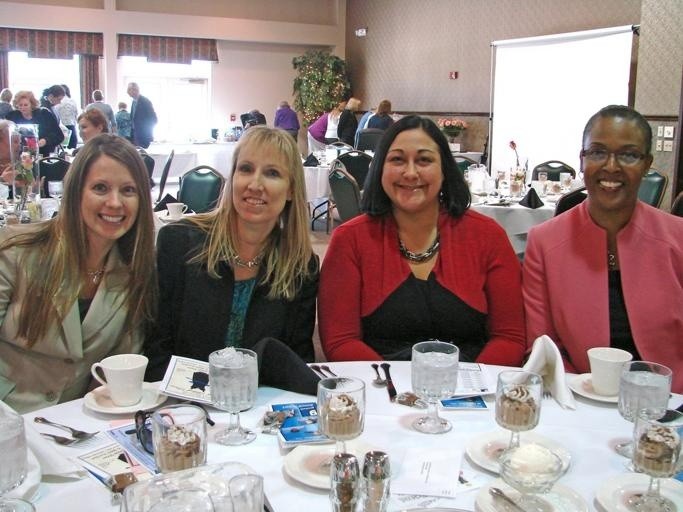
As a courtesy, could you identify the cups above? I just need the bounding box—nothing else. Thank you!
[0,183,59,225]
[121,460,272,511]
[587,345,632,395]
[89,353,148,407]
[151,403,208,473]
[166,202,187,217]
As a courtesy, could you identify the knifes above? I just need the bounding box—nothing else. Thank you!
[379,363,396,402]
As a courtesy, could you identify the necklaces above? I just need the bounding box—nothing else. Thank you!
[607,250,617,269]
[231,250,265,269]
[399,234,440,262]
[77,265,107,285]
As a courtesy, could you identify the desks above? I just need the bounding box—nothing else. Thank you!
[456,174,591,257]
[294,149,375,232]
[150,138,242,179]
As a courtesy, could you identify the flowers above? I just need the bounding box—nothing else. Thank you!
[16,125,39,183]
[509,139,519,171]
[436,118,466,141]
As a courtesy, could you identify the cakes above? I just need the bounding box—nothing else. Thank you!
[319,392,363,441]
[496,386,540,431]
[634,424,683,475]
[156,424,202,473]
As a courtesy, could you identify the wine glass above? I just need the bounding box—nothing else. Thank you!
[316,377,366,453]
[595,359,683,511]
[410,341,458,435]
[47,180,61,210]
[493,367,543,464]
[463,162,584,204]
[0,400,38,511]
[497,438,569,512]
[207,347,258,447]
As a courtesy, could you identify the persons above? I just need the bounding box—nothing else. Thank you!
[318,116,525,366]
[0,81,157,182]
[521,106,683,392]
[0,135,160,414]
[143,124,319,381]
[240,98,395,159]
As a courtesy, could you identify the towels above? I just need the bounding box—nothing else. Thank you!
[24,428,86,482]
[514,335,574,410]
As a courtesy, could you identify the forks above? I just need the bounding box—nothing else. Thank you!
[33,413,100,447]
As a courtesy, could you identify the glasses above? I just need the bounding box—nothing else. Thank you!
[135,410,154,454]
[581,146,647,165]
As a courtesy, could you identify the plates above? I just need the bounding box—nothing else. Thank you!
[466,429,571,483]
[476,480,592,512]
[83,382,169,416]
[281,442,401,489]
[159,217,182,223]
[566,371,620,403]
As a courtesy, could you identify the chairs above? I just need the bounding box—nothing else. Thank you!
[668,192,683,217]
[454,154,479,174]
[150,148,175,205]
[326,150,372,236]
[176,165,225,215]
[532,160,576,183]
[32,156,75,197]
[139,154,155,178]
[352,129,384,151]
[637,167,669,211]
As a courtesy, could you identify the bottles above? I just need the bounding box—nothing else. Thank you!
[362,452,391,510]
[322,450,360,511]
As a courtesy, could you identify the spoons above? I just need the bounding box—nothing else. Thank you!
[370,362,386,384]
